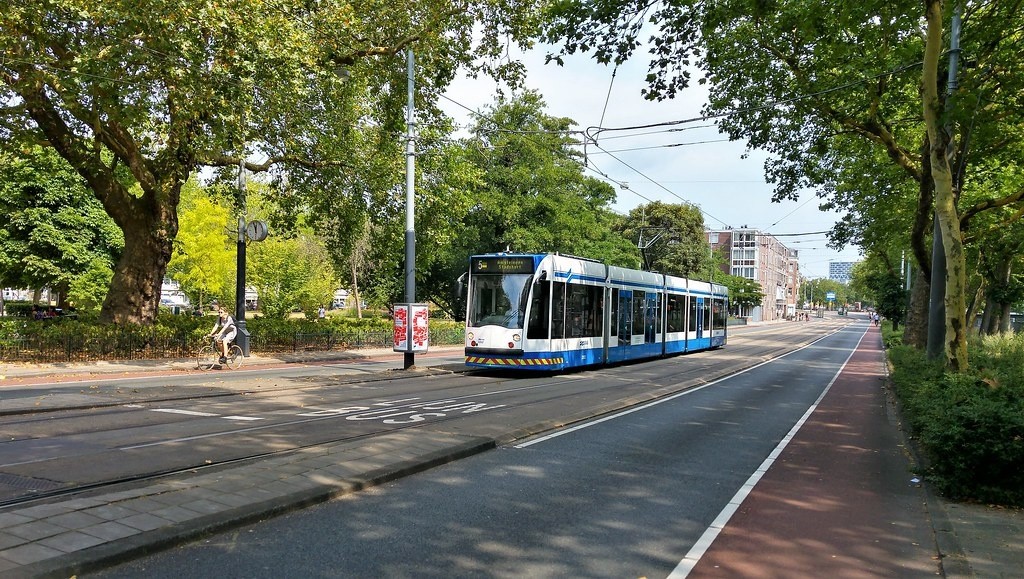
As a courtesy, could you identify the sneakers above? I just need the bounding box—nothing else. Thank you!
[219,357,227,363]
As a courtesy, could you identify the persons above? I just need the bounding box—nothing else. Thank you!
[505,301,523,318]
[250,299,257,310]
[44,306,57,316]
[873,314,879,327]
[32,303,42,321]
[318,304,325,319]
[206,306,238,364]
[551,296,725,344]
[191,306,203,317]
[869,313,872,323]
[387,303,394,321]
[880,315,885,321]
[785,311,810,323]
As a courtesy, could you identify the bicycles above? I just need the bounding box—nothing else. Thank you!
[196,332,243,372]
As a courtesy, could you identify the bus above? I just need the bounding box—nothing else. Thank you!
[456,252,729,371]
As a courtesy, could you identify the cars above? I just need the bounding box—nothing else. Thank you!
[334,303,345,310]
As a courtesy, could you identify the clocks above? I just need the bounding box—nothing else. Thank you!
[246,220,269,241]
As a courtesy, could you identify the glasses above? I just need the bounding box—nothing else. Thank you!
[218,312,224,313]
